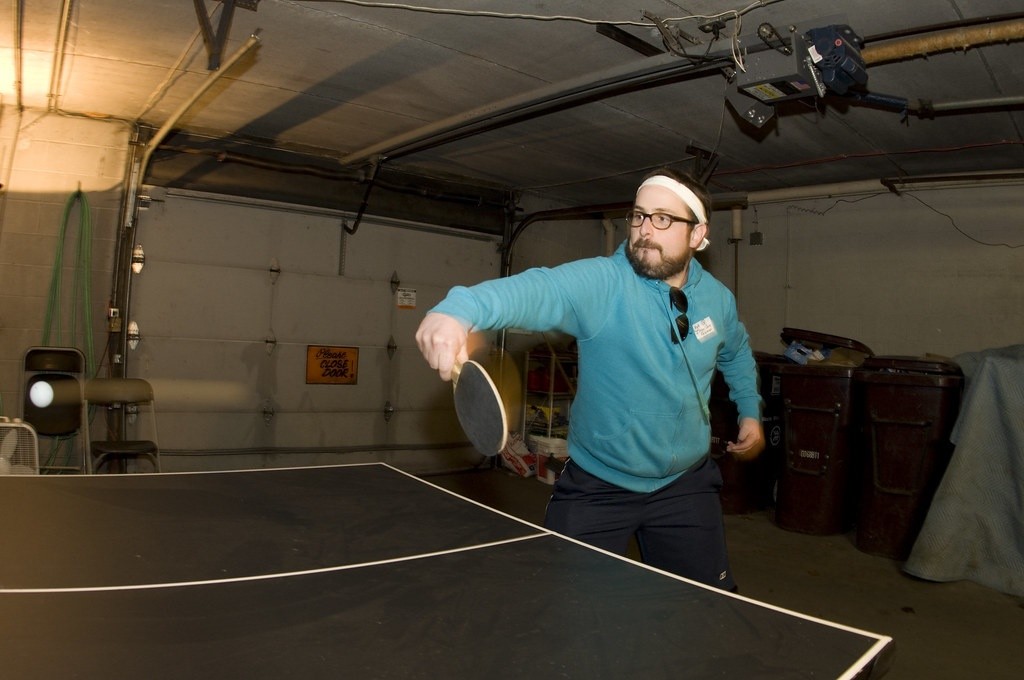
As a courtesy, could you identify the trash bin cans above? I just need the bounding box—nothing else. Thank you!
[744,361,786,507]
[853,356,965,559]
[707,351,788,515]
[774,326,875,537]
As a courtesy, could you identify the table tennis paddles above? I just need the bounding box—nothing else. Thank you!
[450,358,508,457]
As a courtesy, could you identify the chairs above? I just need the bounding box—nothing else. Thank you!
[12,346,93,476]
[84,378,163,475]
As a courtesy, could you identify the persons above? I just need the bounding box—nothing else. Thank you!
[415,166,764,595]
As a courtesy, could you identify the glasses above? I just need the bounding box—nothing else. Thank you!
[669,286,689,344]
[626,210,699,230]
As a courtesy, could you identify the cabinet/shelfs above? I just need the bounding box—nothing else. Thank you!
[522,350,578,440]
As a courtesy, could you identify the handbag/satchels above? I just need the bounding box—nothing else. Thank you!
[499,432,537,478]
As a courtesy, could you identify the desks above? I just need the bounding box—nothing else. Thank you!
[0,460,897,680]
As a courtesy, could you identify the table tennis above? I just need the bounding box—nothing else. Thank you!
[29,381,54,408]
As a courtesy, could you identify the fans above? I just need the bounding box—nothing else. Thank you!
[0,415,40,476]
